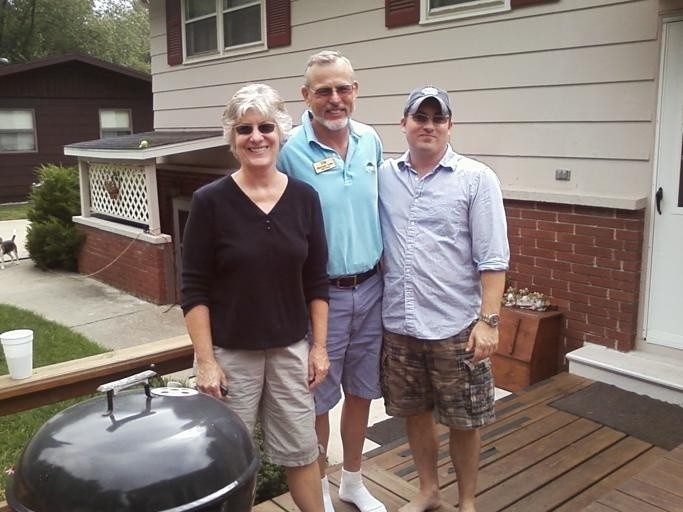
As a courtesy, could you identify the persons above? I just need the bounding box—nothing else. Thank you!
[180,83,331,512]
[273,50,386,512]
[377,86,510,512]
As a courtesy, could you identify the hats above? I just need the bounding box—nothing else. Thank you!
[404,86,452,118]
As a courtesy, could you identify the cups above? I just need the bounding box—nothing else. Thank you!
[0,329,34,379]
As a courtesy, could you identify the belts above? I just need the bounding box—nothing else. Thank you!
[326,263,379,289]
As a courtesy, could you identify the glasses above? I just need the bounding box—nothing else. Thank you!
[236,124,275,134]
[410,113,449,123]
[308,83,355,96]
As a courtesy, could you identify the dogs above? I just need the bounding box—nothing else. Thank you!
[0,235,20,270]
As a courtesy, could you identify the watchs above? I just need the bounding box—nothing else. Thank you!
[478,313,506,328]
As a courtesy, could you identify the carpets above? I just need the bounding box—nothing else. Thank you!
[365,416,408,446]
[548,381,683,450]
